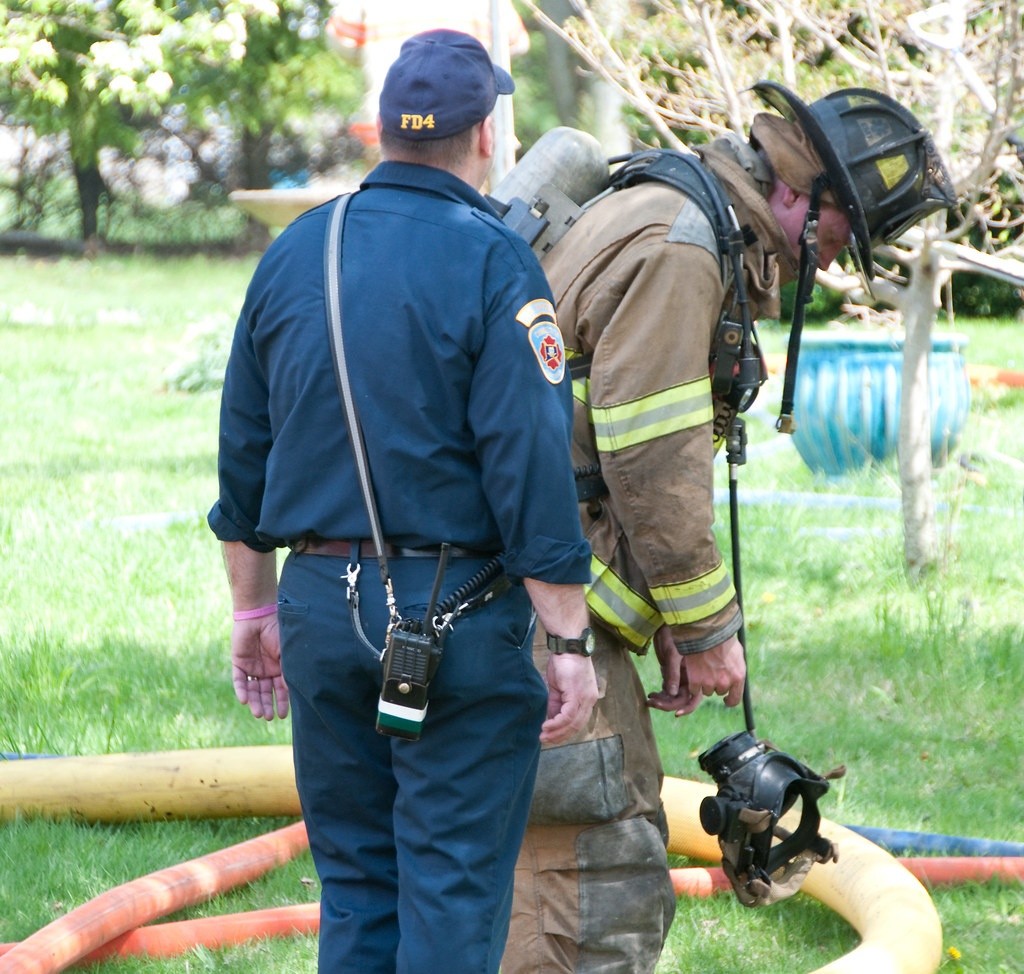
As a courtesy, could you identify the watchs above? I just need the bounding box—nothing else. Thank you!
[546,626,596,657]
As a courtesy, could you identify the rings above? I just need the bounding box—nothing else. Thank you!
[237,675,246,681]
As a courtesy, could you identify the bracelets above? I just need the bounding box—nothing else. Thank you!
[233,603,278,621]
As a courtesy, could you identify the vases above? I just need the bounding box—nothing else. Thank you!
[781,319,978,493]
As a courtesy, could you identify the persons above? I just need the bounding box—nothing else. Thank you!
[206,29,598,974]
[502,87,957,974]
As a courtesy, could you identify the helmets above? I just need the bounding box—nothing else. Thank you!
[752,79,958,279]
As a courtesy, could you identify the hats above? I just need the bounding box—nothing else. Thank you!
[379,29,516,139]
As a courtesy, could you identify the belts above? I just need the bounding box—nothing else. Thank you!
[288,534,506,559]
